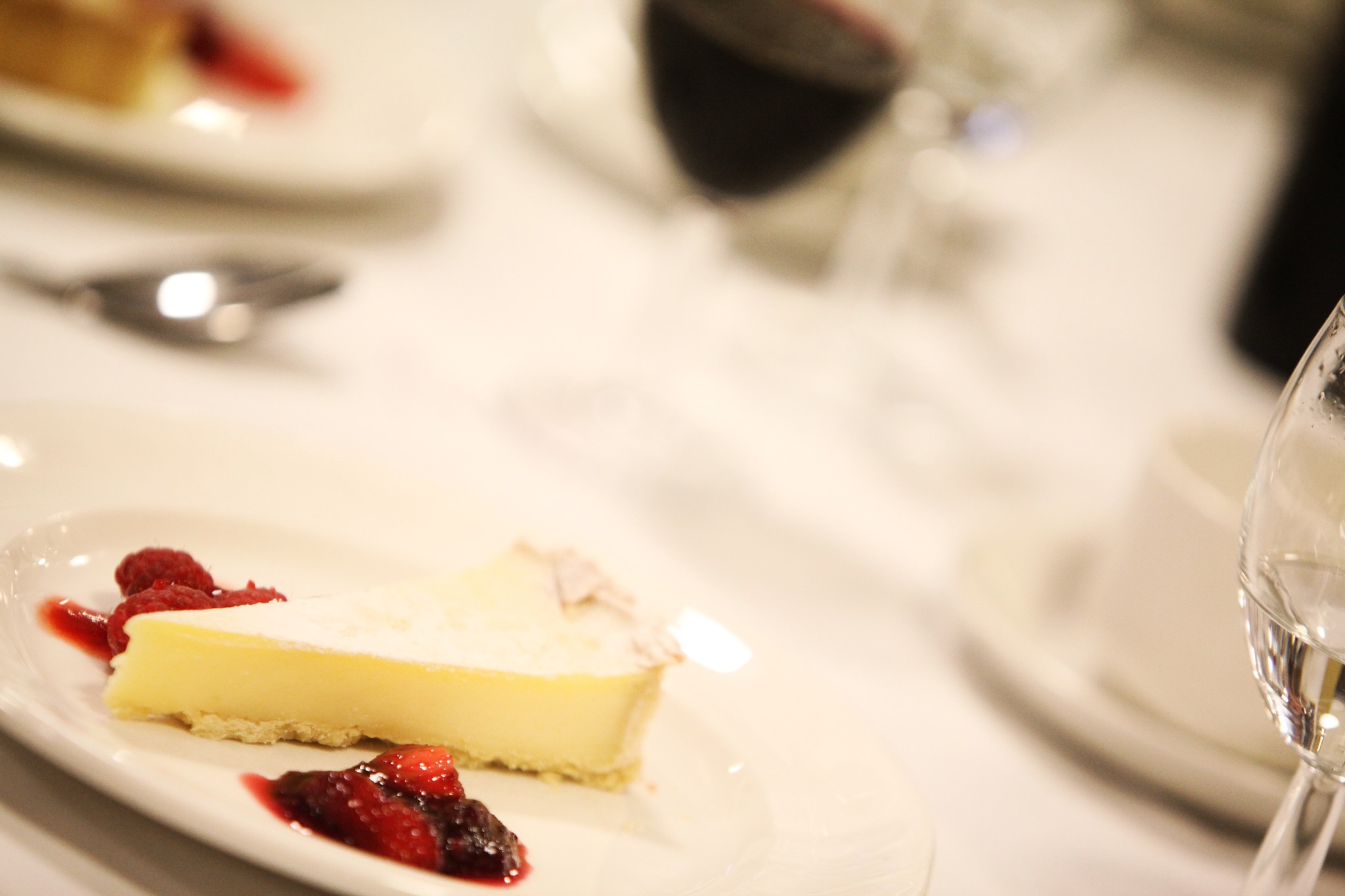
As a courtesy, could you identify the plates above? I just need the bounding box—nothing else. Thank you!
[0,56,446,210]
[948,520,1345,854]
[1,503,938,895]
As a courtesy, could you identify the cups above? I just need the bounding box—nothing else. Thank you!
[1095,422,1345,770]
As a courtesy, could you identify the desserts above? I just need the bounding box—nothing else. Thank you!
[93,549,676,796]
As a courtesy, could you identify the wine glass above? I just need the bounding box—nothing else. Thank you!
[580,0,928,465]
[1229,298,1345,894]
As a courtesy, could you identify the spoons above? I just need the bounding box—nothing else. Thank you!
[0,233,342,341]
